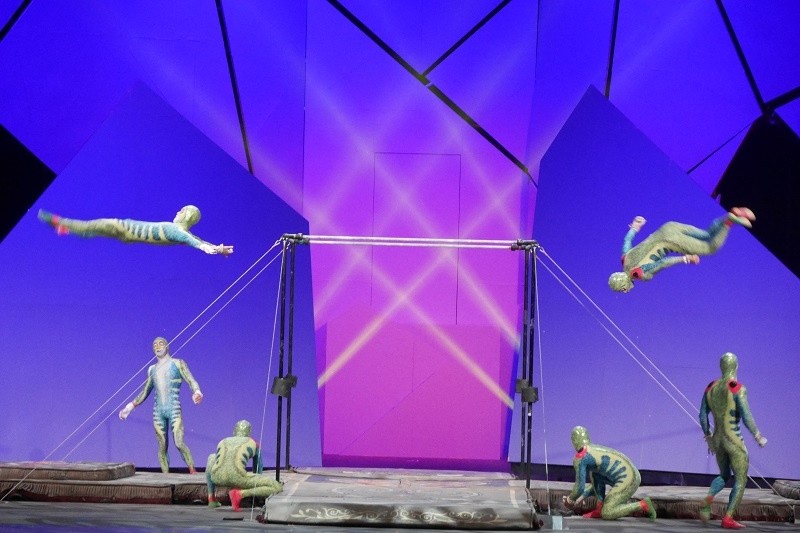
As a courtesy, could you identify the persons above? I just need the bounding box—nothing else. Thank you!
[608,208,756,295]
[118,337,204,475]
[563,425,657,524]
[36,205,234,256]
[698,352,767,529]
[205,420,283,513]
